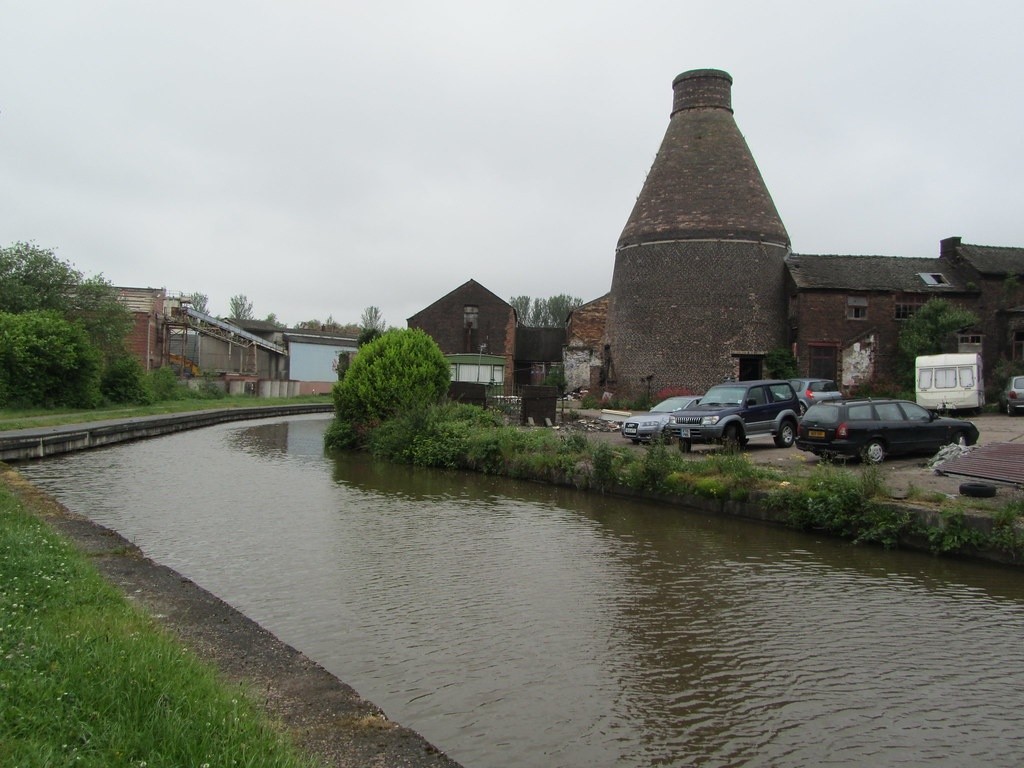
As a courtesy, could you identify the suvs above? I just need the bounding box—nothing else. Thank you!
[665,381,805,453]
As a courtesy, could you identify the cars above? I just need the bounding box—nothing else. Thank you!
[774,379,843,413]
[999,376,1024,416]
[795,397,978,464]
[620,395,720,445]
